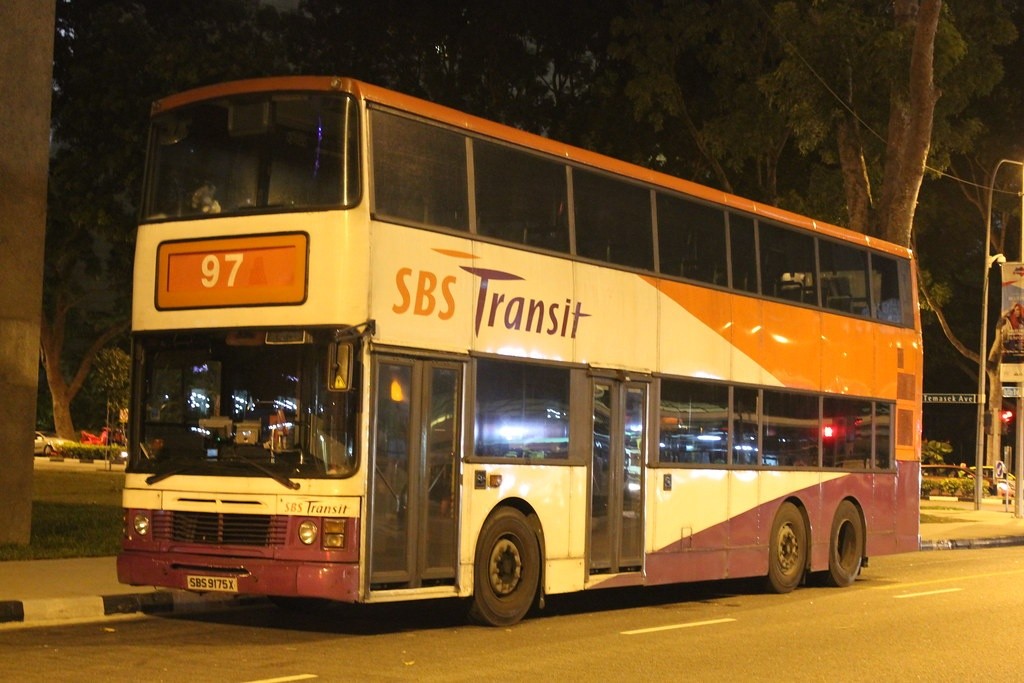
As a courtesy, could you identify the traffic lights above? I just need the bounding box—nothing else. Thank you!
[821,425,836,440]
[1001,410,1015,424]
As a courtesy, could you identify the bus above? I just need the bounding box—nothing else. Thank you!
[112,74,924,627]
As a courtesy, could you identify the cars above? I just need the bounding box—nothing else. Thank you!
[947,465,1016,480]
[34,430,77,457]
[921,465,1015,499]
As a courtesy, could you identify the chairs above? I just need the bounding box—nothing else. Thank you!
[704,260,870,317]
